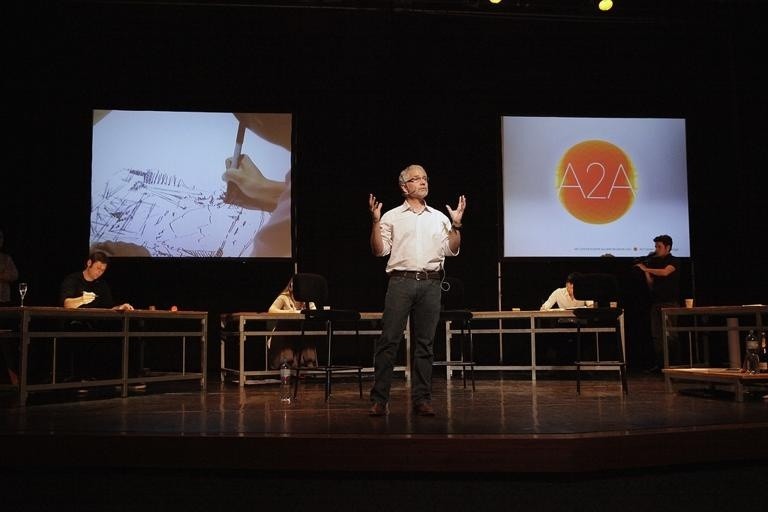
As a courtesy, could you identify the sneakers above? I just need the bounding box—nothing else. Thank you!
[115,378,145,388]
[78,379,89,392]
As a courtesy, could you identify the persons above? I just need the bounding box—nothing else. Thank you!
[633,235,680,376]
[269,275,318,378]
[60,251,149,394]
[540,272,597,366]
[0,254,18,386]
[369,164,467,415]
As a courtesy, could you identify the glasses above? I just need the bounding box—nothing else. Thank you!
[405,176,428,183]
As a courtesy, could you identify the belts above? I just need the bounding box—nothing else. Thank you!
[393,271,440,280]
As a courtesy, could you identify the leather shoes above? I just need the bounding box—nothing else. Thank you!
[368,402,389,416]
[414,404,434,415]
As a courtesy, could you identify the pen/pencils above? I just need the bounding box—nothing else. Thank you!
[226,123,246,203]
[81,292,99,297]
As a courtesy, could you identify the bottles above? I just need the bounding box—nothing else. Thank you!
[280,385,291,407]
[744,328,768,373]
[279,358,291,386]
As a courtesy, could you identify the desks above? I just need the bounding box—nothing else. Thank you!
[445,309,625,382]
[219,311,412,389]
[656,303,767,402]
[1,306,208,407]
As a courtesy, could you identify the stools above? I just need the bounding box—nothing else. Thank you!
[428,308,475,392]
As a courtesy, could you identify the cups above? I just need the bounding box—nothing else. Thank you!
[685,298,693,308]
[609,301,617,309]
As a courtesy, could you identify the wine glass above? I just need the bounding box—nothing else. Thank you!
[19,283,26,308]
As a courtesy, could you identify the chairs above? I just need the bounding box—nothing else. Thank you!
[292,273,365,402]
[572,269,632,395]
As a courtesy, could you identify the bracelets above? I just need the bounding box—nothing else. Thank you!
[451,220,463,233]
